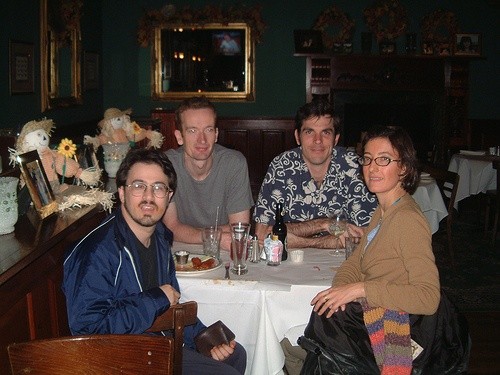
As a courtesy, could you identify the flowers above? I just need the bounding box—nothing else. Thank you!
[314,0,460,51]
[136,2,267,49]
[57,137,77,161]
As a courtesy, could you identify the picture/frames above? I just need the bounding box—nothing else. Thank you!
[18,149,62,219]
[293,29,324,53]
[9,39,36,96]
[453,32,481,55]
[82,52,100,92]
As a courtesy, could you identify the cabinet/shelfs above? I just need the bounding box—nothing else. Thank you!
[0,132,103,375]
[129,111,297,204]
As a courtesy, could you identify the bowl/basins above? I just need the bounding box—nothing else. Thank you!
[289,251,304,262]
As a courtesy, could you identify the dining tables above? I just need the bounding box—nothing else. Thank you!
[171,239,346,375]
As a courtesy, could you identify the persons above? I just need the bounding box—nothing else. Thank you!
[310,122,441,319]
[164,97,255,252]
[60,148,248,375]
[253,100,379,249]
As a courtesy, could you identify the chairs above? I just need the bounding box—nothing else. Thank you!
[483,161,500,244]
[432,169,459,240]
[3,334,174,375]
[148,301,197,375]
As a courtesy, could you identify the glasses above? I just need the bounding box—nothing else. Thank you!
[357,156,401,166]
[125,182,172,198]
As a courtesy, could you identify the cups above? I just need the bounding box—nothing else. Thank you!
[0,177,19,235]
[231,223,251,274]
[345,237,361,260]
[102,143,128,177]
[490,148,495,155]
[201,227,222,260]
[175,250,190,264]
[264,246,283,266]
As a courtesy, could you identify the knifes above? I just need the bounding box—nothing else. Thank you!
[224,260,230,279]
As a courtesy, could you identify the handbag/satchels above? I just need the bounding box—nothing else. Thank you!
[297,336,378,375]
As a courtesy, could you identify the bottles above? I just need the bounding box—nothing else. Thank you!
[344,39,352,54]
[260,202,287,262]
[497,146,500,156]
[250,235,260,263]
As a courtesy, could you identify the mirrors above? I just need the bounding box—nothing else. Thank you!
[40,0,81,112]
[151,18,256,104]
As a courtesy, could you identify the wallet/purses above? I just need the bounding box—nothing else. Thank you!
[196,321,235,358]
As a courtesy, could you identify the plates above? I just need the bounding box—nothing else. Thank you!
[172,254,223,276]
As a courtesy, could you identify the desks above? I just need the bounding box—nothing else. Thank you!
[412,171,449,235]
[448,151,500,212]
[293,52,483,165]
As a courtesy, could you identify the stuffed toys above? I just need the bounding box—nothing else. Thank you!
[13,107,164,212]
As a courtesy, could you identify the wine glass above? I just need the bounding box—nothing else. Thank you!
[328,211,346,256]
